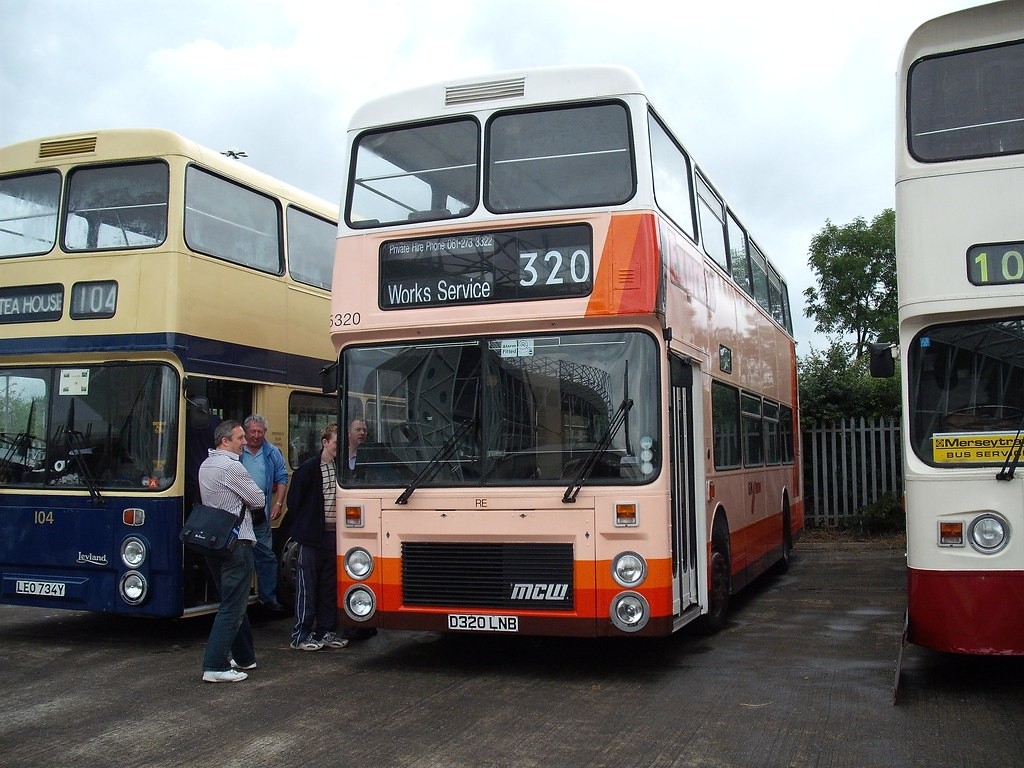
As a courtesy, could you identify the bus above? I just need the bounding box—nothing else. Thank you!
[868,0,1024,658]
[1,126,410,622]
[316,63,805,638]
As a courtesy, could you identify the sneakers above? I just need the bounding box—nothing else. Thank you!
[229,659,257,669]
[290,632,325,651]
[321,630,350,648]
[202,669,248,682]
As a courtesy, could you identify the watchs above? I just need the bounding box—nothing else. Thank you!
[276,501,284,508]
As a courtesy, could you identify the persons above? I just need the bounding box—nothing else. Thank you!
[183,415,377,652]
[199,421,264,682]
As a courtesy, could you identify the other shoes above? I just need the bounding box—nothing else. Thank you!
[258,597,283,611]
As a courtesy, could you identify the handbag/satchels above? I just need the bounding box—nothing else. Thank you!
[179,503,240,557]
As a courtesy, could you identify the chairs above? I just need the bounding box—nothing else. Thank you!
[408,209,452,221]
[459,205,505,216]
